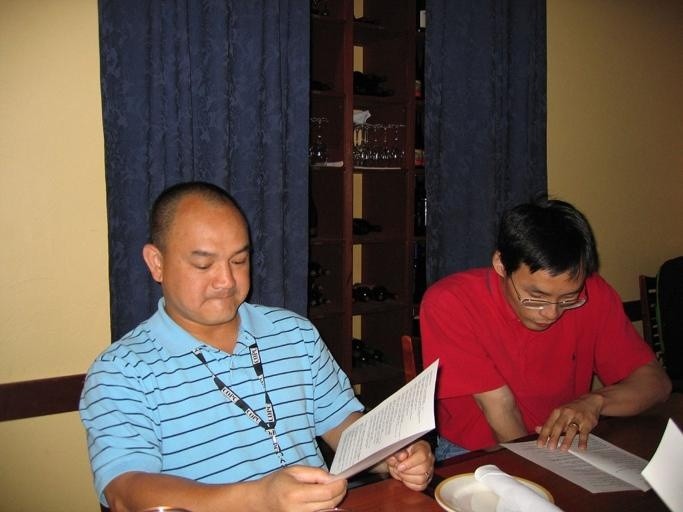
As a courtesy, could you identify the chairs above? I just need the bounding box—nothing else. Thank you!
[401,334,438,455]
[637,274,668,378]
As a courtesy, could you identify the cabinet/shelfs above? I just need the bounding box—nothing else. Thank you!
[308,0,425,483]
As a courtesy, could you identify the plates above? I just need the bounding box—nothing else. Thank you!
[435,472,556,512]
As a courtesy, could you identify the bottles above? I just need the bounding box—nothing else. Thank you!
[309,220,399,368]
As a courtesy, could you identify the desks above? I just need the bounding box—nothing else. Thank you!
[336,391,683,512]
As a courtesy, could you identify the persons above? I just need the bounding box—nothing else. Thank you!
[418,198,675,463]
[77,182,436,512]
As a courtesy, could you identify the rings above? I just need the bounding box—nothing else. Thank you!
[569,422,579,428]
[423,471,432,482]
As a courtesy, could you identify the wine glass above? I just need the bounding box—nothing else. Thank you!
[352,121,404,166]
[309,115,330,164]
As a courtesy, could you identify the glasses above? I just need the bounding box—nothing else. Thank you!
[510,277,588,310]
